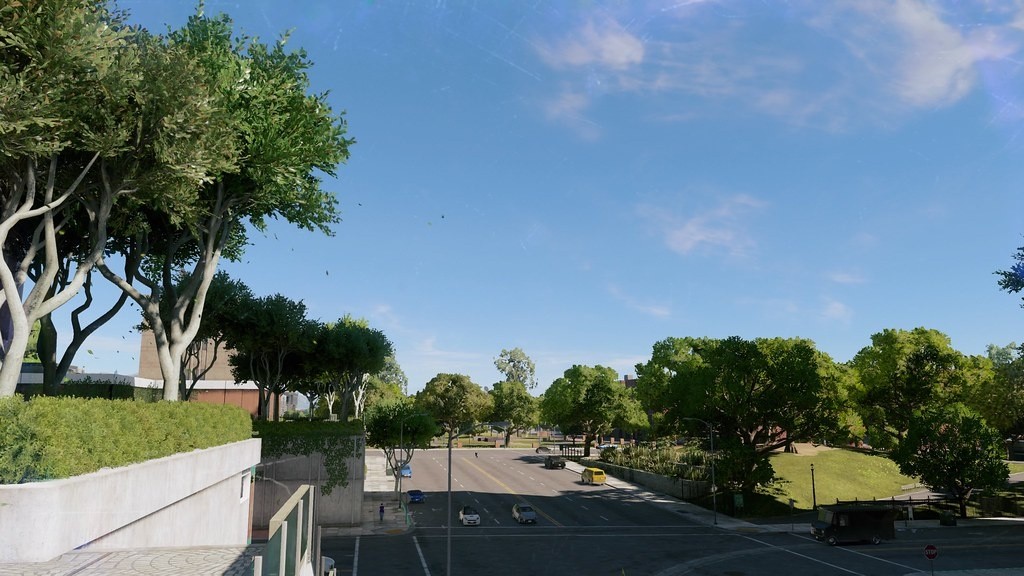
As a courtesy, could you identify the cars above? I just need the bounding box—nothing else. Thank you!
[536,446,551,454]
[407,489,425,504]
[811,504,894,547]
[321,557,338,576]
[459,506,480,526]
[596,444,617,449]
[512,503,538,525]
[394,459,411,479]
[581,467,607,486]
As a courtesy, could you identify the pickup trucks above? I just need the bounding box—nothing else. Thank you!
[545,456,566,469]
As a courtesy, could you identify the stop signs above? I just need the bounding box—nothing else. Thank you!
[923,544,937,560]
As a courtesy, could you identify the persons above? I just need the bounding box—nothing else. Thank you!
[380,503,384,522]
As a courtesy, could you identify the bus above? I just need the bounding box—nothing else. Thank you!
[566,434,586,442]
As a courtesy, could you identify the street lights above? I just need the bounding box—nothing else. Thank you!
[446,420,512,576]
[810,463,818,509]
[682,416,718,524]
